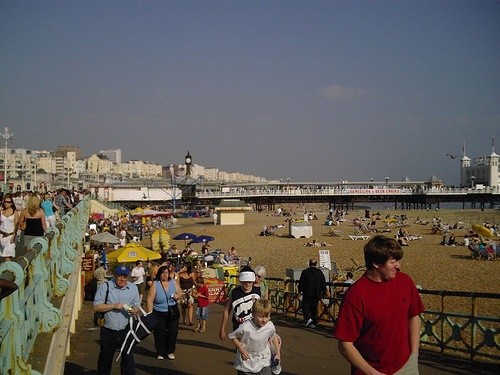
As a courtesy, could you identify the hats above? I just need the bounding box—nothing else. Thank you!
[115,265,130,276]
[239,272,256,282]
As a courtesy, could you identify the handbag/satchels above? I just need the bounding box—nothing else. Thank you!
[115,303,161,362]
[167,303,181,321]
[94,311,106,327]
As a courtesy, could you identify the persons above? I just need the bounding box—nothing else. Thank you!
[297,259,330,326]
[212,212,218,225]
[87,212,177,245]
[304,240,333,247]
[258,225,278,237]
[0,188,90,260]
[82,242,251,375]
[354,209,500,260]
[272,205,349,237]
[186,206,212,219]
[220,266,281,375]
[196,184,496,193]
[336,272,353,297]
[335,234,425,375]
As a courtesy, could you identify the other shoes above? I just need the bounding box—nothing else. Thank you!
[183,321,186,325]
[194,327,200,332]
[166,352,176,360]
[309,323,317,329]
[188,322,195,326]
[156,355,165,360]
[304,318,313,328]
[199,328,207,333]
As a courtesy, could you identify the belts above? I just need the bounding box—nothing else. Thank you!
[0,230,15,238]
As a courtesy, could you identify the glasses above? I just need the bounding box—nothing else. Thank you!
[255,274,261,278]
[4,200,12,203]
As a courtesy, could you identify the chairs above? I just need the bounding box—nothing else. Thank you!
[347,235,371,240]
[331,259,366,282]
[376,228,390,232]
[468,244,496,261]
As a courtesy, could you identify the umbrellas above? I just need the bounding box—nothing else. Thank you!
[172,232,215,246]
[104,231,118,239]
[91,233,119,243]
[471,223,493,237]
[105,243,161,263]
[130,207,169,215]
